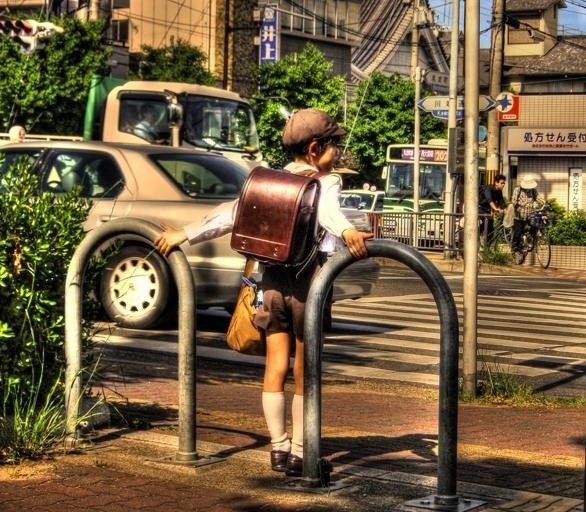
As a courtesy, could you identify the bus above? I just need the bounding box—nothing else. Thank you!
[382,138,502,248]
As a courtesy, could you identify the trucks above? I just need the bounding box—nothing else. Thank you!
[0,75,263,171]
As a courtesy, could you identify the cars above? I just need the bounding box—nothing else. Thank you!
[0,141,379,329]
[339,189,385,232]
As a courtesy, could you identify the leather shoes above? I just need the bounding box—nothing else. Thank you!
[270,450,289,469]
[285,454,333,477]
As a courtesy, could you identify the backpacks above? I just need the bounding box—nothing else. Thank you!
[230,166,333,281]
[478,184,493,202]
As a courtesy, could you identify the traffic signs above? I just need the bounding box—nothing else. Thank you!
[419,96,494,117]
[415,95,497,121]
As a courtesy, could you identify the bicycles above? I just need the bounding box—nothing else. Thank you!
[454,208,516,256]
[509,204,552,268]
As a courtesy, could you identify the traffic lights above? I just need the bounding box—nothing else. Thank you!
[0,20,64,56]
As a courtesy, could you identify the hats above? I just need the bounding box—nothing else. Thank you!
[281,109,346,149]
[520,174,537,191]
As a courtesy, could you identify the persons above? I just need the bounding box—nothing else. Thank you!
[133,103,164,144]
[9,126,26,140]
[478,174,507,249]
[154,107,375,475]
[511,175,550,255]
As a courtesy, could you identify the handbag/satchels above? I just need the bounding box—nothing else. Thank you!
[226,272,288,355]
